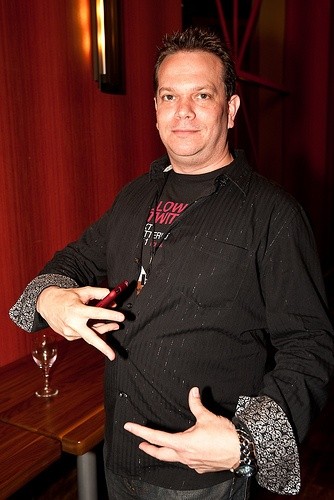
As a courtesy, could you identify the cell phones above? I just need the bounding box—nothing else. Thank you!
[96,280,129,308]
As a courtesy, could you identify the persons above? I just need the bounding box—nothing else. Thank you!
[9,28,334,500]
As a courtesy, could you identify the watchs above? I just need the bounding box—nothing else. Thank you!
[233,421,254,478]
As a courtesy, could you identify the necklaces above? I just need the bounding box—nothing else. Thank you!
[149,195,171,265]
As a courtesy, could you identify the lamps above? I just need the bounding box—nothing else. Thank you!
[90,0,126,94]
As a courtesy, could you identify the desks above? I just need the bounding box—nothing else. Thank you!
[0,340,104,500]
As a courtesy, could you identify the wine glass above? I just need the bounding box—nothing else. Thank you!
[31,334,59,397]
